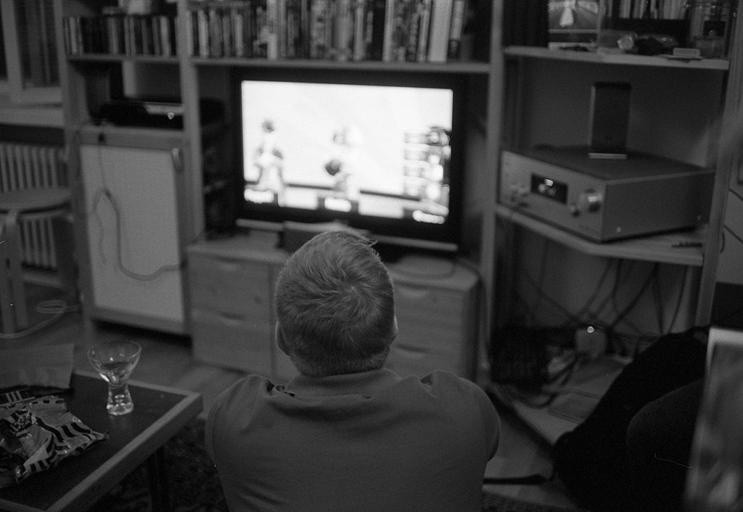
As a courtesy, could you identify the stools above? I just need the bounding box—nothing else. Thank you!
[0,185,72,341]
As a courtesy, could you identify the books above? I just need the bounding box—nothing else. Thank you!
[605,0,729,24]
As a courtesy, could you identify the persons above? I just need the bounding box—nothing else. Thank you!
[254,119,289,204]
[558,0,577,28]
[203,230,503,512]
[318,126,365,214]
[418,124,452,214]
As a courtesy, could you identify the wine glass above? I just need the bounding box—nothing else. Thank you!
[85,341,143,416]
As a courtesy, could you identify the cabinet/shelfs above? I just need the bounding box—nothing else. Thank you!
[55,0,504,75]
[479,1,742,450]
[65,125,203,340]
[184,235,481,387]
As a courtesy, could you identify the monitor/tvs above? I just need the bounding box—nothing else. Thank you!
[230,69,468,267]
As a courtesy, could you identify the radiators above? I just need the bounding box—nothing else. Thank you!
[0,142,68,272]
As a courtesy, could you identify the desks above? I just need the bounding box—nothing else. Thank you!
[0,369,204,512]
